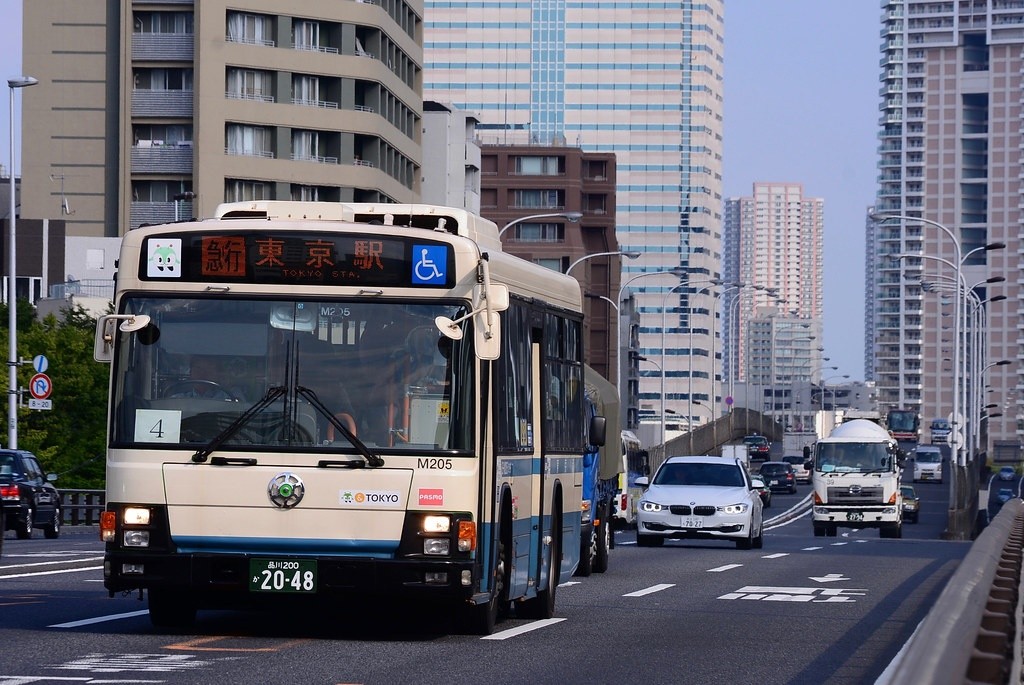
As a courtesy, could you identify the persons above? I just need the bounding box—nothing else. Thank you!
[168,355,245,404]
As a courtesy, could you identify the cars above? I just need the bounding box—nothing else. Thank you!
[996,465,1016,503]
[750,475,771,508]
[910,446,945,484]
[929,419,951,444]
[742,435,772,462]
[0,449,64,541]
[757,461,798,494]
[781,456,812,484]
[634,455,764,549]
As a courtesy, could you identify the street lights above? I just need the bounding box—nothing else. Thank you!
[630,279,725,461]
[8,77,39,450]
[664,281,860,454]
[870,213,1013,509]
[583,269,686,400]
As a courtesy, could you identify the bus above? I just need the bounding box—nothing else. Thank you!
[613,430,650,530]
[93,201,585,635]
[885,410,920,442]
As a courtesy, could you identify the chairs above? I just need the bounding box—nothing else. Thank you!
[826,449,851,466]
[935,424,939,429]
[712,469,741,486]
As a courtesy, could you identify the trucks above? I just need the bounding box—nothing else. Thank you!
[572,363,624,578]
[803,419,905,538]
